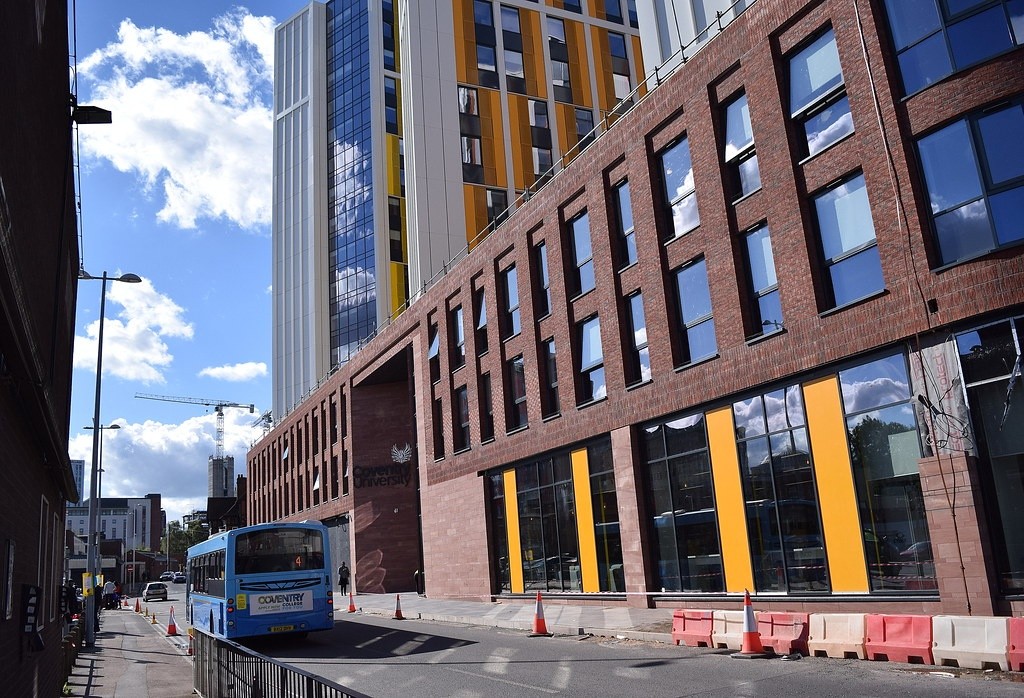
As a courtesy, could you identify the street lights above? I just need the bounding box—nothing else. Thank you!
[75,269,142,648]
[83,423,121,586]
[133,503,143,592]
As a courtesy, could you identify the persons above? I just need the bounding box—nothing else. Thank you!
[103,578,117,610]
[93,576,102,632]
[113,581,122,609]
[338,562,350,596]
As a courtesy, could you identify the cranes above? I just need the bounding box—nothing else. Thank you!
[133,391,255,460]
[252,409,272,438]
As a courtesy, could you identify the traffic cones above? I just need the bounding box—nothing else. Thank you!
[134,598,139,612]
[730,590,773,659]
[138,605,143,614]
[165,606,181,637]
[527,591,554,637]
[775,566,786,591]
[348,593,357,612]
[123,597,129,606]
[151,612,157,624]
[392,594,406,619]
[186,634,194,656]
[143,607,149,617]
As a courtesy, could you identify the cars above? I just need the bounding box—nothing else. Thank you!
[172,573,186,584]
[523,557,575,580]
[899,541,933,560]
[558,557,578,578]
[762,529,902,583]
[160,572,174,581]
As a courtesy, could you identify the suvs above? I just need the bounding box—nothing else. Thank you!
[142,582,167,602]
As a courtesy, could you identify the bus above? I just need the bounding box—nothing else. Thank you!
[594,498,820,593]
[188,519,334,641]
